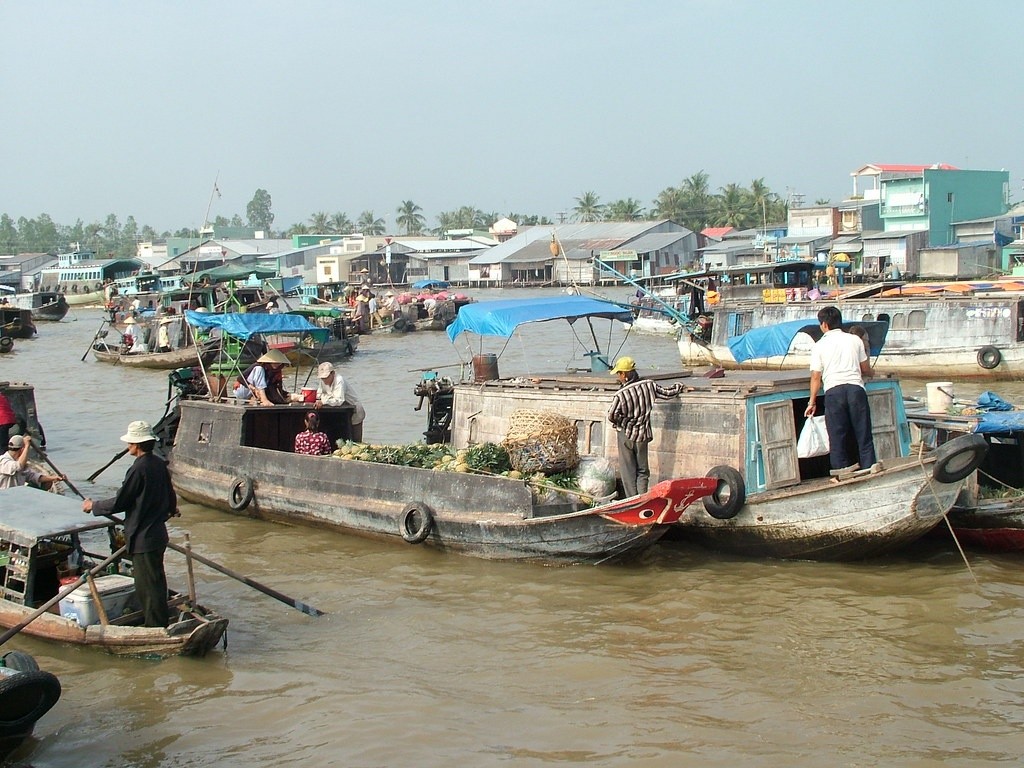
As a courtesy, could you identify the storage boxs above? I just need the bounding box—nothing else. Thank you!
[58,574,135,627]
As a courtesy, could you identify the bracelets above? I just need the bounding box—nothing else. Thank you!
[808,402,816,406]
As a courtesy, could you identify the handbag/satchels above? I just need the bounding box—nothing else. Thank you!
[796,415,829,458]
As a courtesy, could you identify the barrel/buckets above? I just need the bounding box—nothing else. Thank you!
[926,382,955,414]
[302,388,317,401]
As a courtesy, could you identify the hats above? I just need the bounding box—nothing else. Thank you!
[360,268,369,272]
[385,291,393,297]
[160,317,172,325]
[362,285,370,289]
[256,348,291,363]
[609,356,637,374]
[317,362,334,378]
[8,435,26,448]
[265,302,274,309]
[120,421,159,443]
[123,316,137,324]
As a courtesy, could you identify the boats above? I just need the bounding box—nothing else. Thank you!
[901,382,1024,553]
[664,257,1024,386]
[0,648,62,762]
[407,296,987,563]
[0,275,472,371]
[0,485,230,658]
[163,307,719,568]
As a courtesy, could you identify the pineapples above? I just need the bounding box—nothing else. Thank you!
[332,438,594,505]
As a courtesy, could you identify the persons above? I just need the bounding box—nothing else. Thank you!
[368,294,383,328]
[804,306,876,479]
[266,302,282,314]
[0,392,17,455]
[353,294,369,330]
[124,317,144,343]
[0,435,67,490]
[0,298,9,305]
[294,410,331,456]
[608,357,686,497]
[361,286,369,296]
[158,316,173,352]
[383,291,400,310]
[313,362,366,442]
[420,298,436,317]
[82,420,179,628]
[132,297,141,310]
[232,348,290,406]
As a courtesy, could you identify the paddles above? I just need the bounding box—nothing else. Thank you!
[86,448,129,481]
[0,543,127,646]
[29,438,85,500]
[107,514,326,616]
[81,321,106,361]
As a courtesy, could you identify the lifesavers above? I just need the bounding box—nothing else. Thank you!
[977,346,1001,370]
[394,318,406,329]
[0,650,40,673]
[0,336,12,347]
[924,433,989,483]
[398,500,434,544]
[701,465,745,520]
[432,313,443,320]
[227,475,254,512]
